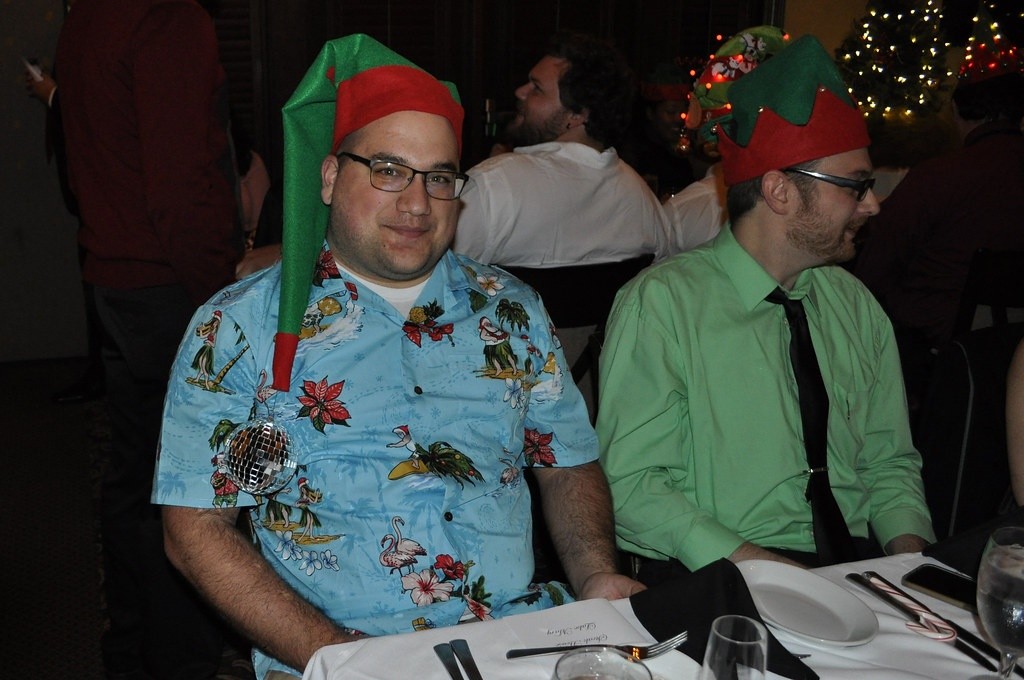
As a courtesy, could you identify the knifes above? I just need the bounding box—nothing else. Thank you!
[861,570,1024,680]
[843,573,998,672]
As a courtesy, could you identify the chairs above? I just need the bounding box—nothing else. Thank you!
[487,253,656,431]
[901,245,1024,540]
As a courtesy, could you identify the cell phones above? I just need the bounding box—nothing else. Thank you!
[901,563,978,614]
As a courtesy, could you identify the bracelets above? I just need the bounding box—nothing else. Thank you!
[44,79,56,105]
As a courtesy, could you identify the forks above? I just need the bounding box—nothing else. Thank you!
[505,630,688,660]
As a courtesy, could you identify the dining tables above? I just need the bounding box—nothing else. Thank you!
[299,505,1024,680]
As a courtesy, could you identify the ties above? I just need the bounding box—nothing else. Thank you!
[764,287,856,571]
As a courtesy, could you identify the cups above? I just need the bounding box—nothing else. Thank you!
[552,646,653,680]
[698,614,768,680]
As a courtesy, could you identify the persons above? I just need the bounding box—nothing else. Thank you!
[852,0,1024,426]
[150,31,648,680]
[595,34,937,680]
[623,55,702,202]
[232,97,272,252]
[54,0,247,680]
[452,39,678,268]
[22,46,142,680]
[237,173,285,278]
[0,0,116,680]
[912,244,1024,542]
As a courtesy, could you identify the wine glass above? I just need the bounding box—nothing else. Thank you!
[975,526,1024,680]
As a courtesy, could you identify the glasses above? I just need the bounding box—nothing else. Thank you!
[335,149,469,201]
[788,166,876,201]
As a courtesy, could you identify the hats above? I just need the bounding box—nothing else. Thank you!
[272,30,466,393]
[710,32,873,186]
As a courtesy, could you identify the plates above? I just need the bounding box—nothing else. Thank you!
[734,558,880,650]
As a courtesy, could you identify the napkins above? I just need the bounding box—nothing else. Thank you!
[629,557,821,680]
[923,509,1024,579]
